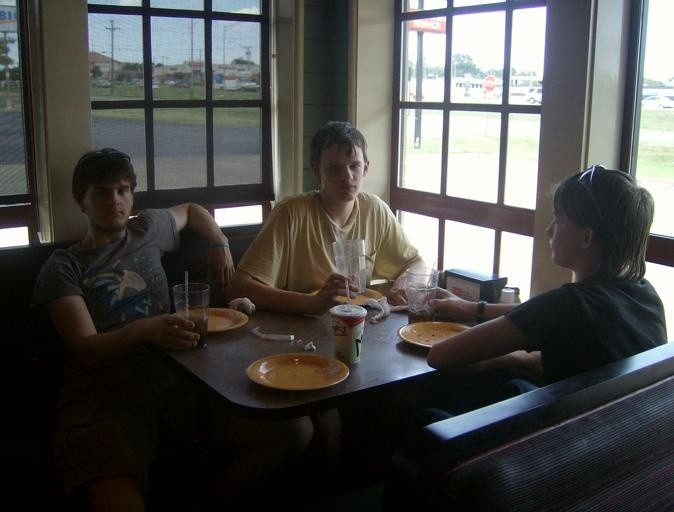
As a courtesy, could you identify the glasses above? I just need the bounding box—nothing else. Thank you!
[579,164,602,224]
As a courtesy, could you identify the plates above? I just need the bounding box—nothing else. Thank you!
[313,285,380,310]
[184,307,252,330]
[248,353,351,389]
[398,320,469,349]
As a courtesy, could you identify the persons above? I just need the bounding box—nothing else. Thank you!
[32,149,236,511]
[222,121,431,464]
[424,163,665,422]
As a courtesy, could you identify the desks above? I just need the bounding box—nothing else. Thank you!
[166,310,479,422]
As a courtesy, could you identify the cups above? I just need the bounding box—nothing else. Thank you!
[331,306,368,365]
[333,238,366,294]
[173,283,210,348]
[403,269,437,322]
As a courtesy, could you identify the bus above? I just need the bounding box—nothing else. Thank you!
[505,74,544,95]
[448,77,503,100]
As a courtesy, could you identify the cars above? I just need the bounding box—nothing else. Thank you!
[1,79,21,89]
[93,73,263,90]
[639,93,674,111]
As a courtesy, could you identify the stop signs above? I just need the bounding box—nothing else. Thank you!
[484,76,496,90]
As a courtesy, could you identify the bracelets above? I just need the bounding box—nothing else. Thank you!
[477,301,487,322]
[208,242,230,247]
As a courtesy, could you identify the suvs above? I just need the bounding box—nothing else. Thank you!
[524,87,542,104]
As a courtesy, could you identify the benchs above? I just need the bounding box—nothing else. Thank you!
[420,342,673,511]
[0,224,264,512]
